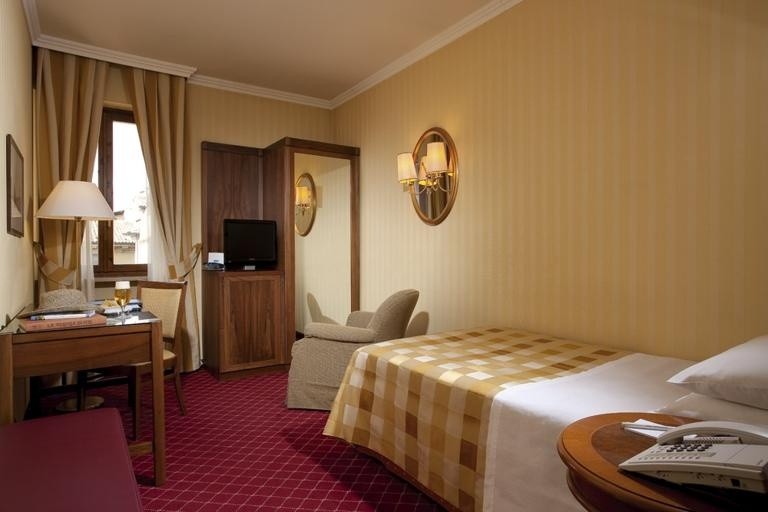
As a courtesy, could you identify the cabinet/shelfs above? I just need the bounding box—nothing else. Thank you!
[262,136,358,364]
[201,270,283,380]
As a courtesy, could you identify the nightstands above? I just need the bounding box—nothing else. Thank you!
[556,412,768,512]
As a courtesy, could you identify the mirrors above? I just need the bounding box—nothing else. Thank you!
[295,173,317,236]
[409,127,458,226]
[290,149,356,361]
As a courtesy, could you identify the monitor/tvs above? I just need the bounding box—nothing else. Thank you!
[223,217,278,272]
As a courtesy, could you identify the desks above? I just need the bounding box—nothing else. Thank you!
[0,307,166,486]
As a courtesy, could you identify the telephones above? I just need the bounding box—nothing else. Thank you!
[618,421,768,494]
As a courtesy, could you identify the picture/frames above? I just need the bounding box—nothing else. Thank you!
[5,134,24,238]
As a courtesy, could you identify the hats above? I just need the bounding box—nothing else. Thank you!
[16,288,105,319]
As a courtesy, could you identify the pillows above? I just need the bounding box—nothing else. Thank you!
[667,335,768,410]
[653,392,768,427]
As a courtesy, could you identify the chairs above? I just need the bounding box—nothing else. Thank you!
[286,288,419,411]
[78,280,188,440]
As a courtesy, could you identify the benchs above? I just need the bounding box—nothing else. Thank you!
[0,408,142,511]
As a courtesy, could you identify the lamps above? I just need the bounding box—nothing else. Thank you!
[397,142,447,195]
[34,180,118,411]
[418,156,454,192]
[295,187,309,215]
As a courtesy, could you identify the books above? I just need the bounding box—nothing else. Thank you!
[624,418,697,444]
[18,298,143,333]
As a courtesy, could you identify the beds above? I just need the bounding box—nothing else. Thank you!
[323,326,768,512]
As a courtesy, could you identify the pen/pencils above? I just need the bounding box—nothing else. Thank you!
[620,422,675,431]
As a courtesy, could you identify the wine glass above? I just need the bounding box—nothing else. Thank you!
[113,279,134,320]
